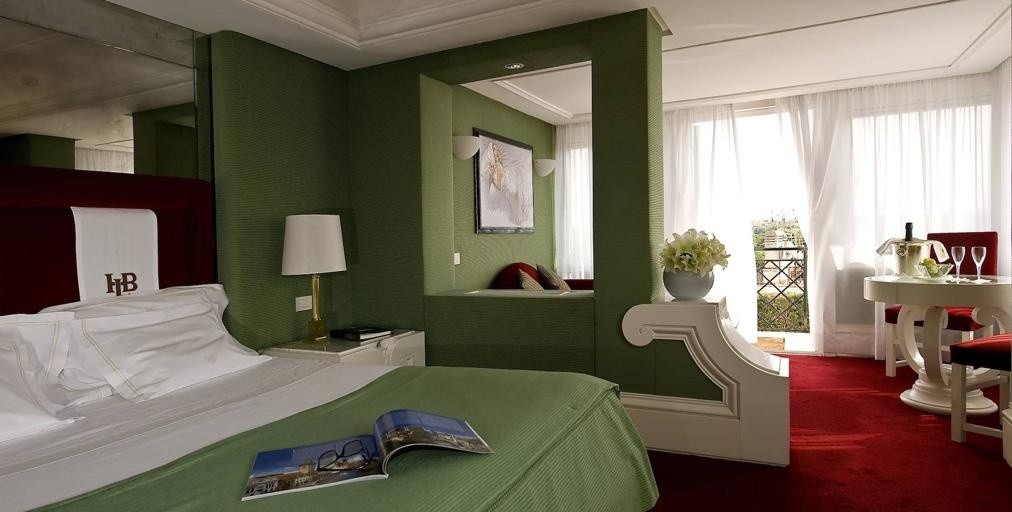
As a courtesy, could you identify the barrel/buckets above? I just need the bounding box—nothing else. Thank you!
[896,242,929,276]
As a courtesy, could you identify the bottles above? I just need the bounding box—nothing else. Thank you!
[903,222,915,243]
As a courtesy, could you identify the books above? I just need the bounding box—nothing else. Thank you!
[240,410,497,502]
[330,325,393,342]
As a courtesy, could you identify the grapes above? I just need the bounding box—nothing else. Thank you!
[923,257,942,278]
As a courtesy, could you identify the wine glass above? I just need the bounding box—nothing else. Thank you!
[970,247,987,282]
[951,245,966,283]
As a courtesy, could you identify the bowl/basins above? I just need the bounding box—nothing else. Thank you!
[894,243,933,277]
[915,264,953,280]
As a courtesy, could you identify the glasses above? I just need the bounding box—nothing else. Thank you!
[317,440,370,471]
